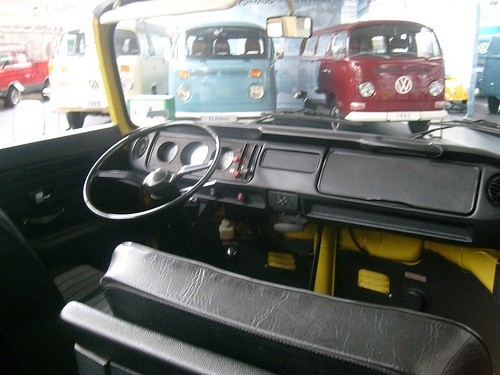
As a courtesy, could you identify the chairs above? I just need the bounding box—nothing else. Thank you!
[127,38,140,52]
[212,37,230,55]
[0,205,116,364]
[58,242,498,374]
[385,37,413,54]
[244,39,261,54]
[191,40,208,56]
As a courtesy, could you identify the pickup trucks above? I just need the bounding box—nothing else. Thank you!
[0,42,50,108]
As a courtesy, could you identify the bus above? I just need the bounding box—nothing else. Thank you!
[47,23,175,130]
[294,20,446,137]
[166,19,284,125]
